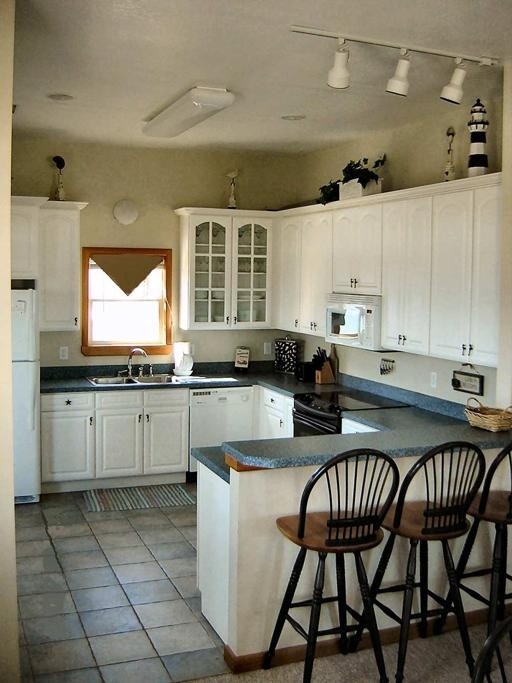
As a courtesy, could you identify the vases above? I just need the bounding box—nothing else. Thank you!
[337,177,384,200]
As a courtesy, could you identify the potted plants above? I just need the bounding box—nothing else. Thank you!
[316,154,386,206]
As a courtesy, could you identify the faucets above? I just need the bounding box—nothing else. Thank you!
[117,348,153,378]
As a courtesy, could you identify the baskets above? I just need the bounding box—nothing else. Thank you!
[464,396,512,433]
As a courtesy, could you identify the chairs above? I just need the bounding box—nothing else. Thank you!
[263,447,399,683]
[351,442,486,683]
[432,440,512,677]
[470,615,511,683]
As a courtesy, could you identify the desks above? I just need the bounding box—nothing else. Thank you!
[190,403,512,676]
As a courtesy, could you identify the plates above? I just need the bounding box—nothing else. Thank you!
[173,369,193,376]
[240,295,264,299]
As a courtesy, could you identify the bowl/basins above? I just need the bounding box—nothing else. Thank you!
[214,315,224,321]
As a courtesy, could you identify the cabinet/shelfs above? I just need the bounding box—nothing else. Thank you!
[95,390,143,478]
[189,214,274,330]
[275,216,303,334]
[302,211,331,338]
[331,205,381,295]
[429,189,473,364]
[258,386,287,439]
[144,389,189,476]
[470,181,499,368]
[40,391,96,484]
[38,207,81,333]
[381,196,432,355]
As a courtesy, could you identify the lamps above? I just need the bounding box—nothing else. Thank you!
[142,85,235,139]
[326,50,351,89]
[439,67,466,105]
[385,58,411,97]
[113,199,138,224]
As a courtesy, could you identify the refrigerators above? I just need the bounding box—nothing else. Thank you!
[10,279,41,505]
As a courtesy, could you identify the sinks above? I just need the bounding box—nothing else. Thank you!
[85,376,137,386]
[135,374,183,384]
